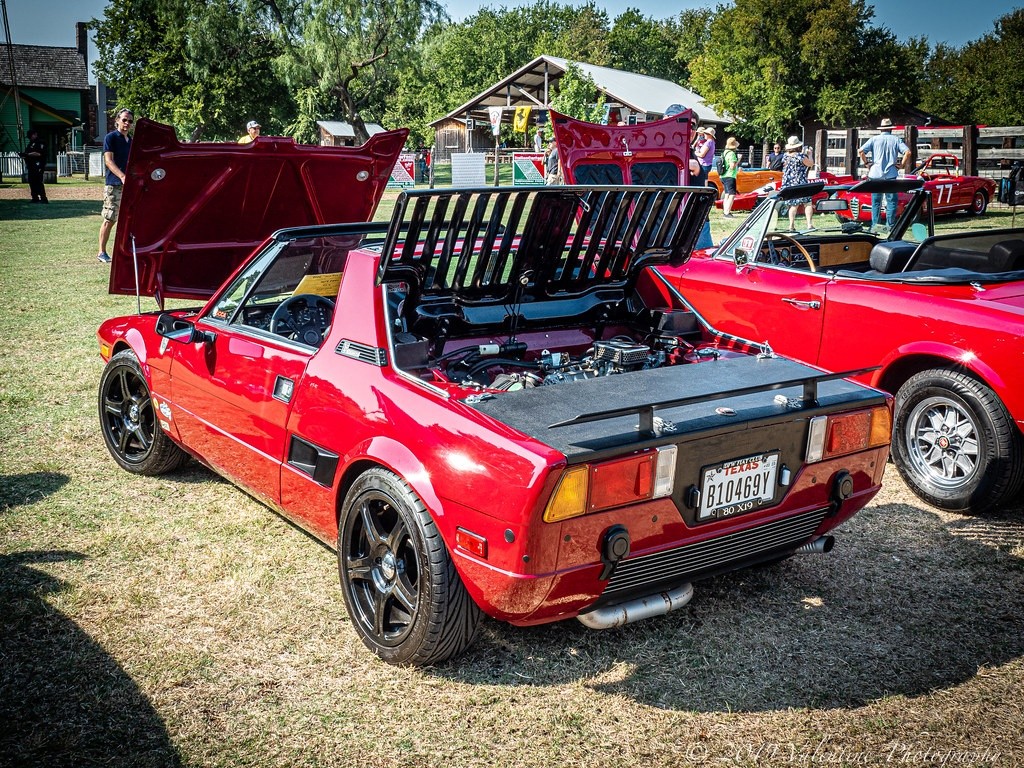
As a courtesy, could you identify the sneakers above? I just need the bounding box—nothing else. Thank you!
[97,252,111,262]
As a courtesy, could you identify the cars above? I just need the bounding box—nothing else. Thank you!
[830,153,997,227]
[705,162,785,202]
[95,119,894,668]
[548,109,1024,516]
[715,172,861,217]
[999,165,1024,206]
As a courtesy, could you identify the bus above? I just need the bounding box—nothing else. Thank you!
[820,125,988,175]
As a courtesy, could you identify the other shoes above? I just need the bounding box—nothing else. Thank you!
[723,213,734,218]
[29,199,49,203]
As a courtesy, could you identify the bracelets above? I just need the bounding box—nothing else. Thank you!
[558,171,560,172]
[865,162,868,165]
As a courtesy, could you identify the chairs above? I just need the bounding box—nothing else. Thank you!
[980,239,1024,273]
[865,240,919,278]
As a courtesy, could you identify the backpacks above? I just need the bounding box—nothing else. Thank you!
[716,151,731,175]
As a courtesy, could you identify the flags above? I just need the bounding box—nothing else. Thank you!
[489,106,502,136]
[514,105,530,134]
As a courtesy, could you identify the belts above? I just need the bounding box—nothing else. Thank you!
[549,172,557,174]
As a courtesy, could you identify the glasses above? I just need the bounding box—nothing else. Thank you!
[252,127,259,130]
[691,123,698,130]
[775,148,779,149]
[122,118,133,124]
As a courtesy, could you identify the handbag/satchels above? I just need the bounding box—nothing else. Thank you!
[998,160,1024,206]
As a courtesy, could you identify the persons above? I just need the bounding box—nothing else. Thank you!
[239,120,261,143]
[18,129,48,204]
[541,137,561,186]
[502,138,508,148]
[98,108,134,262]
[767,144,783,172]
[781,136,815,231]
[858,118,911,232]
[695,127,716,187]
[533,131,542,152]
[663,104,713,249]
[692,127,707,152]
[418,154,429,183]
[719,137,742,218]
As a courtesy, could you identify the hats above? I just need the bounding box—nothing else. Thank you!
[703,127,716,139]
[725,137,739,149]
[247,121,261,129]
[664,104,688,116]
[696,127,705,133]
[785,136,803,149]
[877,118,896,129]
[547,137,555,141]
[25,129,36,138]
